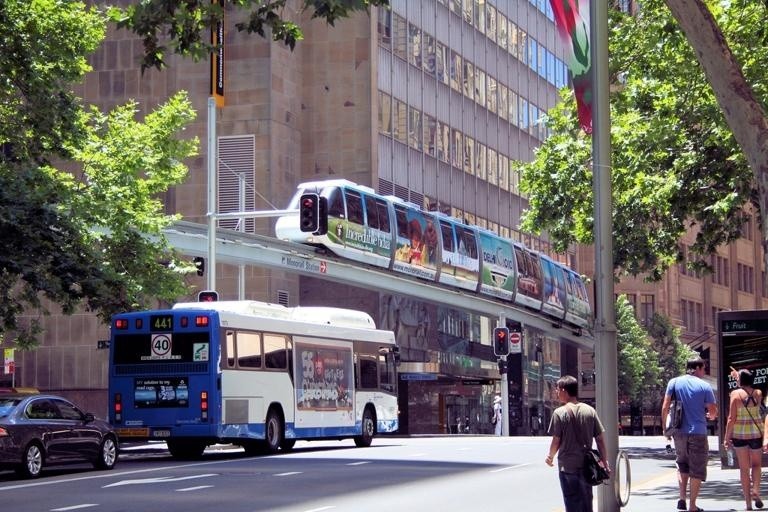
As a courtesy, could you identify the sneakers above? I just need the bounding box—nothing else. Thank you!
[677,500,685,510]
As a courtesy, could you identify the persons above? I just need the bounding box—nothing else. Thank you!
[547,277,564,309]
[494,396,502,435]
[545,375,611,512]
[724,366,764,511]
[531,413,538,435]
[409,219,438,267]
[661,356,717,512]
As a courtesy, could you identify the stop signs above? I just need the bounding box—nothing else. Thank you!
[509,332,521,353]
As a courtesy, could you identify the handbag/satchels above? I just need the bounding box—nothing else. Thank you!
[662,401,681,436]
[583,451,608,486]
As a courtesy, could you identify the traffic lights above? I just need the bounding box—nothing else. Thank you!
[197,291,218,303]
[493,328,509,356]
[193,257,204,276]
[299,193,318,233]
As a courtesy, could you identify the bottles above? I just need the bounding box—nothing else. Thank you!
[726,447,734,467]
[336,214,345,239]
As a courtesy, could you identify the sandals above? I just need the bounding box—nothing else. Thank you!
[751,494,762,508]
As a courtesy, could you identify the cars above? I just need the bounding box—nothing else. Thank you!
[0,386,49,420]
[0,393,119,478]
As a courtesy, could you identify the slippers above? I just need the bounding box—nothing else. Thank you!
[695,507,702,512]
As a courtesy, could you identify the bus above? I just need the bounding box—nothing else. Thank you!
[107,299,401,459]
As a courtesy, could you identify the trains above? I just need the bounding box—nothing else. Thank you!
[275,177,593,330]
